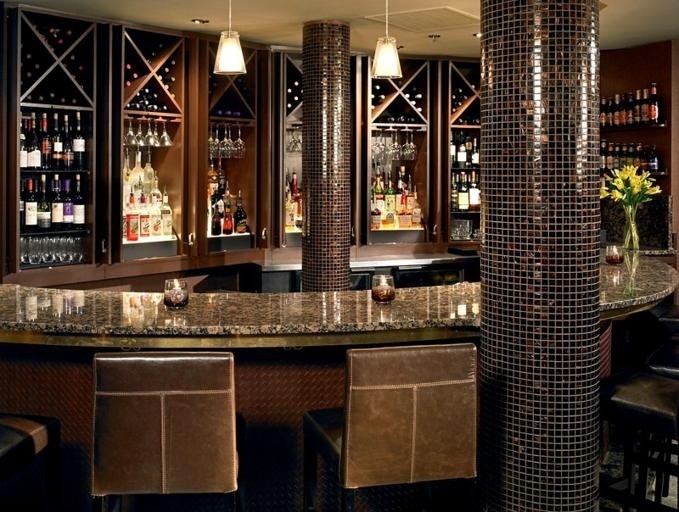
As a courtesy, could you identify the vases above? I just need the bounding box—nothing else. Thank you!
[622,205,639,251]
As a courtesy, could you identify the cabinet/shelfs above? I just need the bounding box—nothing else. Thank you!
[276,48,305,247]
[367,55,428,243]
[10,4,103,270]
[115,23,188,260]
[451,59,479,238]
[202,38,261,252]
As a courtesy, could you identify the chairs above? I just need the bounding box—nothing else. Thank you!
[91,351,237,507]
[302,342,477,507]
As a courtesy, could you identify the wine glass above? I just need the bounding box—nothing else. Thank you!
[384,130,401,159]
[144,119,154,145]
[161,119,173,146]
[125,121,138,147]
[287,127,302,152]
[371,129,384,160]
[234,123,246,157]
[402,129,417,160]
[220,123,235,157]
[153,119,161,146]
[135,119,146,145]
[208,121,220,159]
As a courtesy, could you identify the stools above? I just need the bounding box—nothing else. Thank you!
[649,336,676,374]
[608,370,678,512]
[0,414,58,506]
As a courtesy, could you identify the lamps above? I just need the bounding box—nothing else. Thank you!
[212,2,245,74]
[369,1,403,77]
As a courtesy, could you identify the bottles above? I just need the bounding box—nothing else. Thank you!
[373,63,425,125]
[126,38,182,112]
[211,187,253,235]
[22,22,92,106]
[368,161,424,231]
[448,169,482,214]
[20,111,88,170]
[123,147,174,242]
[598,138,662,175]
[18,173,91,233]
[601,81,660,127]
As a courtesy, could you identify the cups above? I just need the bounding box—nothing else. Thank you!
[451,220,469,240]
[21,236,84,264]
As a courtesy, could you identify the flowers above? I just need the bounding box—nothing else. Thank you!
[600,164,662,205]
